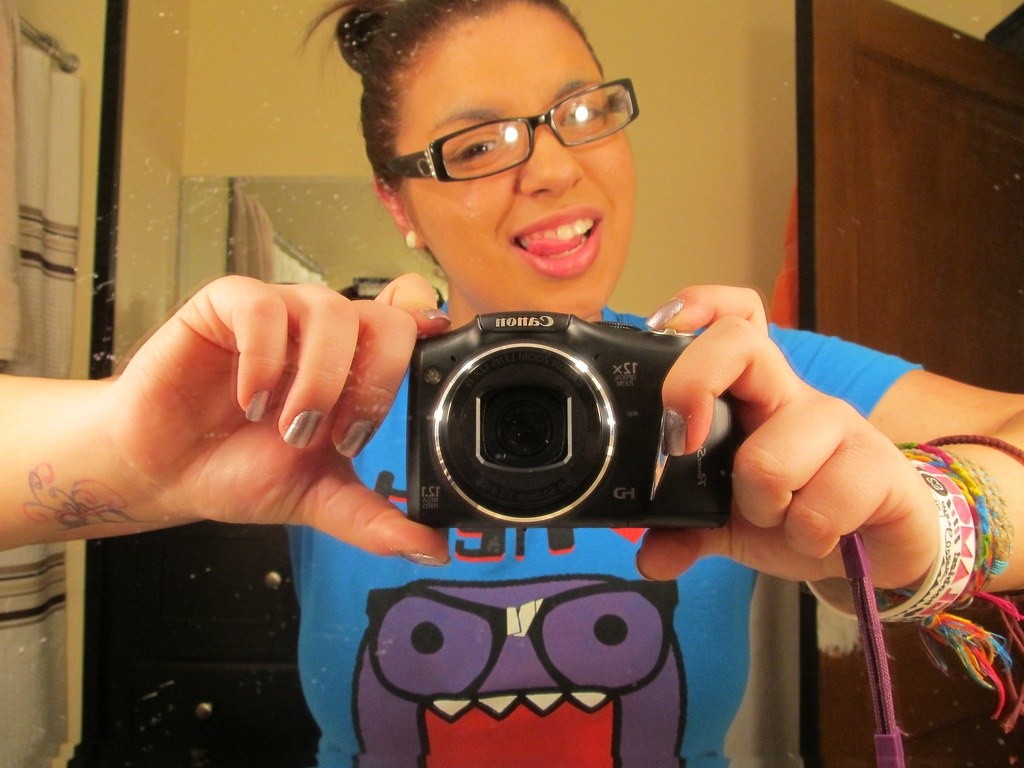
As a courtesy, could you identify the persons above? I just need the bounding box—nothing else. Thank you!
[1,0,1024,768]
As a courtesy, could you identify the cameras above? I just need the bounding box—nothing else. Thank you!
[405,311,741,528]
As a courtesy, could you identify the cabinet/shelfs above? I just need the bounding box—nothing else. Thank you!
[84,523,322,768]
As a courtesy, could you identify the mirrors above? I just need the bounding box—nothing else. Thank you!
[89,1,448,380]
[177,176,449,317]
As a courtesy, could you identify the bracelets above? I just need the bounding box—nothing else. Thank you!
[808,433,1024,736]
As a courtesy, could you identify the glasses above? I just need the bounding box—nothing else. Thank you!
[390,78,640,182]
